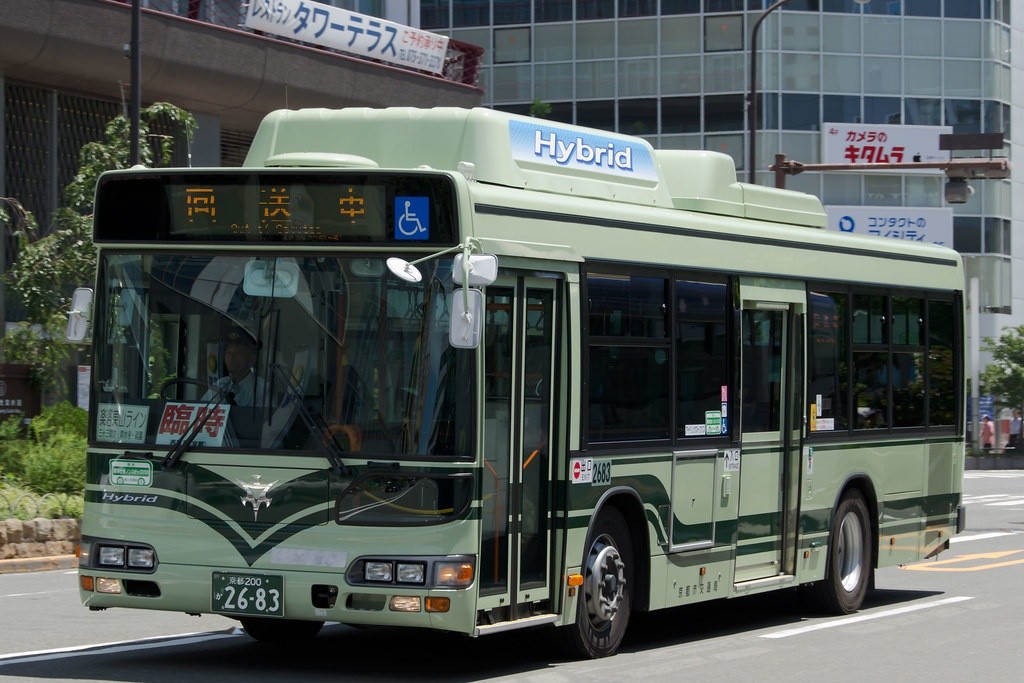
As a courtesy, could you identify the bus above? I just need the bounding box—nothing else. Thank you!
[584,274,841,434]
[63,106,968,660]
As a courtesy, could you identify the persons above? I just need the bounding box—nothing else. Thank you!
[1005,410,1024,453]
[877,353,901,387]
[200,324,287,409]
[979,413,995,453]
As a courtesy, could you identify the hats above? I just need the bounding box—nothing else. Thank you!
[223,327,262,351]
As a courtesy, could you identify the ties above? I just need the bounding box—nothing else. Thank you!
[226,391,235,405]
